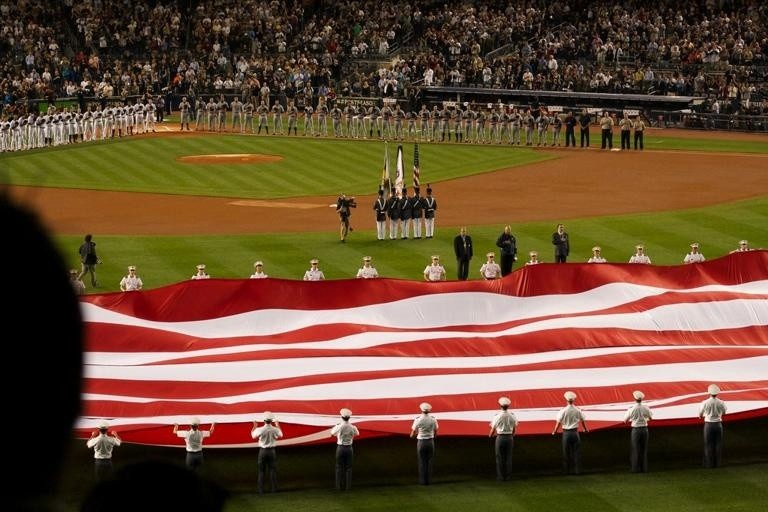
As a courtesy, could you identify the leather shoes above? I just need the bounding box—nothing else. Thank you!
[342,240,345,243]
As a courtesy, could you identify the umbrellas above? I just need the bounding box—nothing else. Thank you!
[378,139,392,192]
[412,142,422,195]
[393,145,407,196]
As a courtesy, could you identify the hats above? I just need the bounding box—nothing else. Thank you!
[378,183,432,194]
[363,250,538,261]
[498,397,511,407]
[69,259,319,275]
[592,240,748,252]
[263,412,274,420]
[191,417,200,425]
[633,390,644,400]
[564,391,577,402]
[707,383,720,396]
[419,402,432,412]
[340,408,352,418]
[98,421,108,429]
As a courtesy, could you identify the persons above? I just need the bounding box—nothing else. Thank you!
[409,402,439,485]
[623,389,655,474]
[480,252,502,280]
[599,112,614,149]
[250,411,285,493]
[0,95,165,150]
[178,96,592,148]
[119,265,145,291]
[523,250,544,265]
[454,227,473,279]
[619,112,634,150]
[488,395,518,481]
[495,224,518,276]
[78,233,103,287]
[683,243,706,265]
[551,391,591,476]
[627,245,652,264]
[588,247,609,263]
[81,463,228,510]
[698,384,726,468]
[634,115,646,149]
[165,88,173,115]
[0,191,84,511]
[172,418,216,467]
[187,91,196,121]
[423,254,445,282]
[728,240,762,254]
[399,189,414,239]
[249,260,270,279]
[192,263,211,279]
[68,265,84,296]
[303,259,326,280]
[411,188,424,239]
[357,256,379,278]
[372,190,388,241]
[388,187,401,240]
[0,1,765,112]
[422,188,437,238]
[86,424,124,480]
[337,194,356,242]
[329,406,362,492]
[552,224,570,262]
